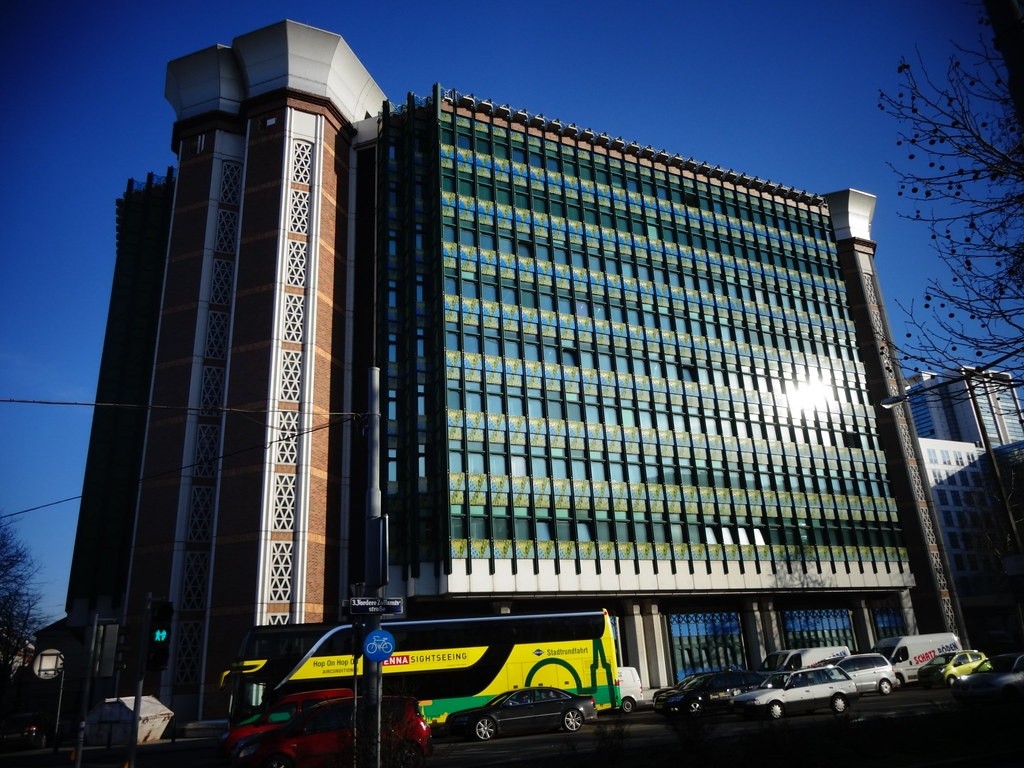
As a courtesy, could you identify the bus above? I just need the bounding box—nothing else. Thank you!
[216,606,622,739]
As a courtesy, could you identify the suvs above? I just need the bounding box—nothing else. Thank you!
[218,688,353,757]
[726,664,858,721]
[808,651,899,695]
[227,696,435,768]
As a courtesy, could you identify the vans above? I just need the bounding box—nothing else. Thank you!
[616,666,644,714]
[869,631,963,689]
[758,645,852,674]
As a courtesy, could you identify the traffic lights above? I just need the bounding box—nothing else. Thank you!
[148,599,175,667]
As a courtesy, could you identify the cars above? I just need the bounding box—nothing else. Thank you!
[918,649,994,691]
[444,686,598,741]
[0,707,62,749]
[950,652,1024,708]
[652,669,765,720]
